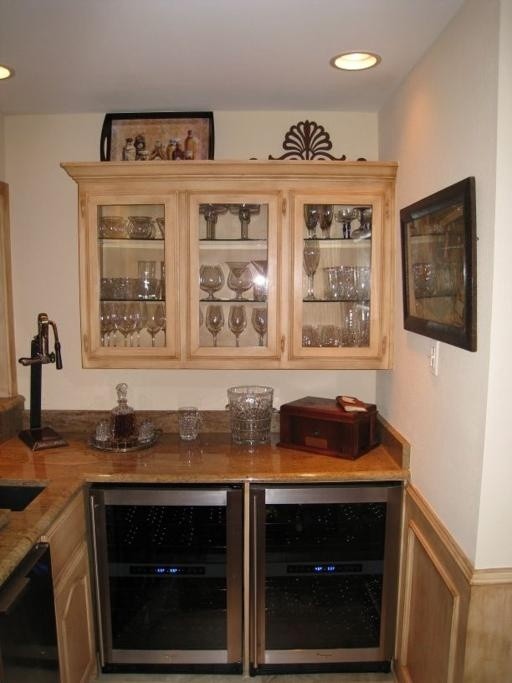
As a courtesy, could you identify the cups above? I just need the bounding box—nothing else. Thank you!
[321,326,342,347]
[177,406,200,441]
[138,261,165,300]
[325,265,369,300]
[100,278,136,300]
[227,386,274,446]
[156,217,165,239]
[303,326,321,347]
[342,303,369,347]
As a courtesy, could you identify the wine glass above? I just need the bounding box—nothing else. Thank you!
[303,240,322,301]
[227,304,248,346]
[199,264,225,301]
[251,306,268,346]
[304,204,333,239]
[206,304,225,348]
[354,205,370,238]
[99,302,166,347]
[336,207,355,239]
[225,260,266,302]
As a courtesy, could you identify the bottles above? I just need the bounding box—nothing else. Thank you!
[110,382,137,441]
[128,214,154,238]
[99,216,124,238]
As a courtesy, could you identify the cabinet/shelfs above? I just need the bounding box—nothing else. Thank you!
[36,491,101,683]
[287,161,399,370]
[61,159,287,370]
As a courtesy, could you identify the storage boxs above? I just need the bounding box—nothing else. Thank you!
[276,396,381,461]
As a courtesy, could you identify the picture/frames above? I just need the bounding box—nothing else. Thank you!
[400,174,479,353]
[99,110,217,161]
